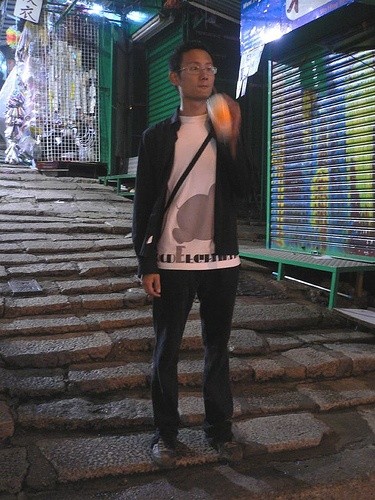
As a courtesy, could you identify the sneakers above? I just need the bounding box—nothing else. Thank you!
[152,439,178,466]
[217,436,243,463]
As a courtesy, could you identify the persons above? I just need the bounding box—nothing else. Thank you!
[130,41,255,469]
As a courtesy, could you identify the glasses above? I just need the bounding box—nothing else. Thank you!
[175,65,217,75]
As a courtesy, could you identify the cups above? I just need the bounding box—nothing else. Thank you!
[206,94,234,140]
